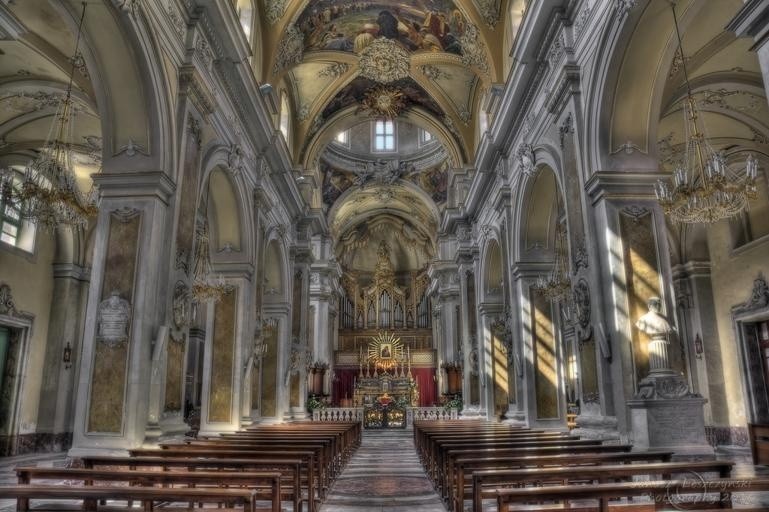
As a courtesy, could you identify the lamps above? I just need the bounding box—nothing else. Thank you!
[654,3,759,224]
[0,3,107,230]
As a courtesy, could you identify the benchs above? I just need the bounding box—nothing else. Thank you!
[1,419,363,511]
[413,419,768,510]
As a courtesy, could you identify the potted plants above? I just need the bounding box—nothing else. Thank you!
[444,400,464,413]
[307,397,329,413]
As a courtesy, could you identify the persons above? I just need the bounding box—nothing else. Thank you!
[308,1,450,55]
